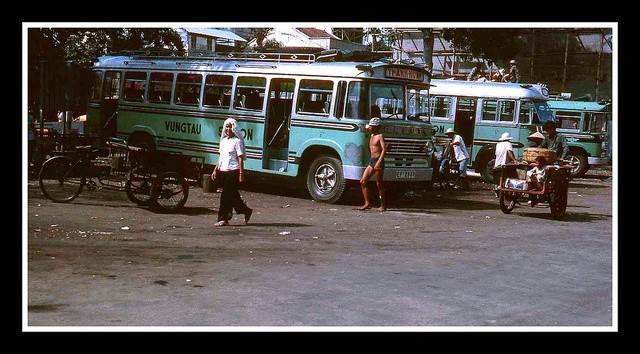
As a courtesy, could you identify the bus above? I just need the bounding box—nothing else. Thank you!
[84,50,438,203]
[546,94,611,178]
[376,78,559,183]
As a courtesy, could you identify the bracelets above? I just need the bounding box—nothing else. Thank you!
[240,171,244,173]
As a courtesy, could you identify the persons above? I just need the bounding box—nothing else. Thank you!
[57,110,63,122]
[357,117,386,211]
[527,132,545,147]
[529,155,545,190]
[211,117,253,225]
[538,121,569,160]
[445,127,471,190]
[508,59,519,82]
[467,63,488,81]
[493,132,516,197]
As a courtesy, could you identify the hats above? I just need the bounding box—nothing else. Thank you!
[368,117,380,126]
[499,133,513,141]
[445,129,457,134]
[528,132,545,140]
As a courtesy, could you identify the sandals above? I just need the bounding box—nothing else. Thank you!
[245,208,253,223]
[214,221,228,226]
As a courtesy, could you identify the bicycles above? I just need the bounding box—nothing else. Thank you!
[38,145,123,202]
[439,142,460,188]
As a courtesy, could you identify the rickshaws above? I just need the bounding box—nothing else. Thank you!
[106,137,205,213]
[495,156,575,219]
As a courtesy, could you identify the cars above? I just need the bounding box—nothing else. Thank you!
[34,123,57,136]
